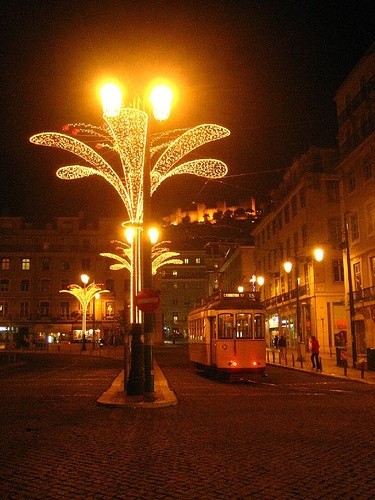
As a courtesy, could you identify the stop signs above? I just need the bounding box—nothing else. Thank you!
[139,289,161,314]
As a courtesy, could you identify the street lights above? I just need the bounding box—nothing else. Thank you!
[283,254,301,341]
[26,77,231,403]
[313,213,358,368]
[58,273,110,351]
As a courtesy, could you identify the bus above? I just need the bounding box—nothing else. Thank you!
[186,290,268,384]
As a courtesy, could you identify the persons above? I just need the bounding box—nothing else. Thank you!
[311,336,321,371]
[279,335,286,359]
